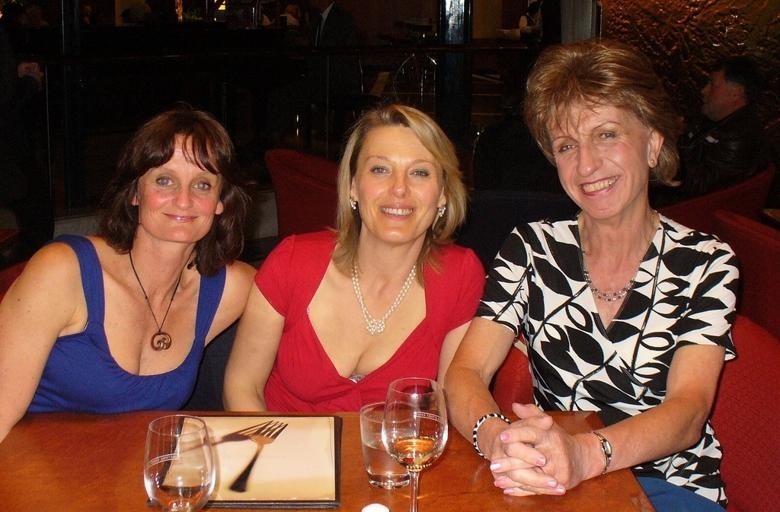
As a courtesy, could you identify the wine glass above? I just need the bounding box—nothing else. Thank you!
[143,413,214,511]
[382,379,451,512]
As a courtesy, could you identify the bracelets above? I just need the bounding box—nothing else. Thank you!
[592,430,616,479]
[472,412,513,460]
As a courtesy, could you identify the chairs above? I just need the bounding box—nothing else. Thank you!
[0,259,34,303]
[264,148,341,240]
[488,315,780,512]
[712,208,780,335]
[655,159,778,232]
[455,188,580,275]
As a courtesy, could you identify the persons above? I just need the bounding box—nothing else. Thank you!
[440,35,749,512]
[22,3,53,32]
[220,100,485,413]
[0,60,46,252]
[652,55,770,202]
[306,1,359,144]
[262,3,303,29]
[1,98,259,444]
[519,2,539,42]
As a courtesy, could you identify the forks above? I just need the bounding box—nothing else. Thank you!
[229,422,285,494]
[205,420,283,446]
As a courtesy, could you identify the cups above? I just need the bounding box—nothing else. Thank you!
[358,400,410,490]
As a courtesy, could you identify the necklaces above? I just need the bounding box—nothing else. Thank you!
[349,252,419,336]
[129,247,183,352]
[581,207,658,302]
